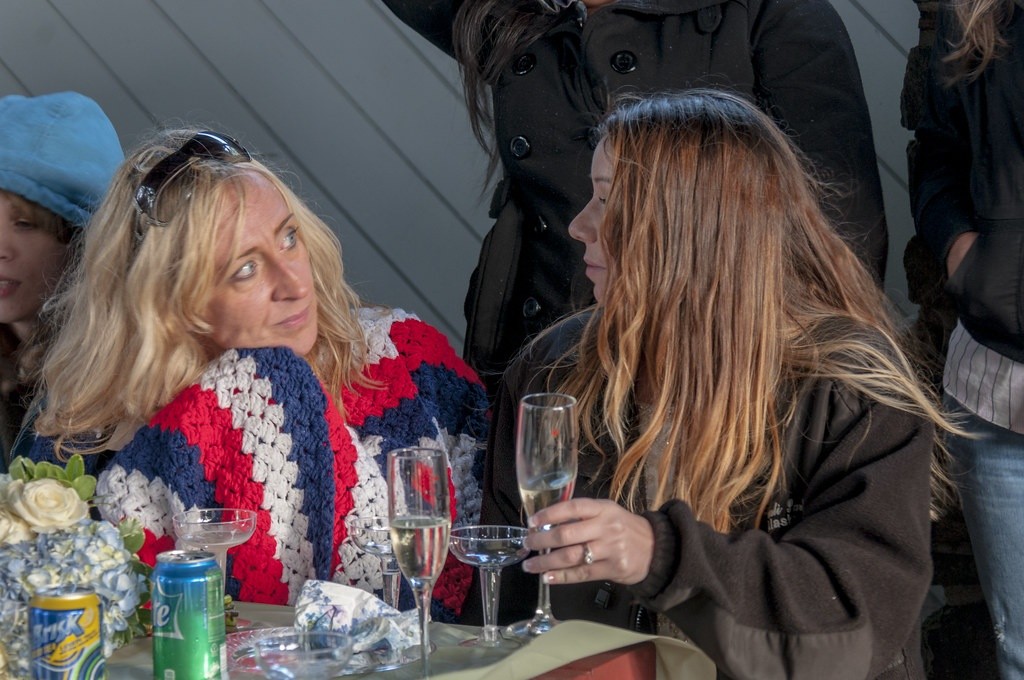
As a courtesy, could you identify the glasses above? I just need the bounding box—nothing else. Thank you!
[127,130,252,269]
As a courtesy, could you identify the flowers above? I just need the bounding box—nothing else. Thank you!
[0,452,154,680]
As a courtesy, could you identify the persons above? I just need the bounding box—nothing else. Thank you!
[0,90,132,519]
[9,122,490,624]
[900,0,1024,680]
[481,72,997,680]
[383,1,888,633]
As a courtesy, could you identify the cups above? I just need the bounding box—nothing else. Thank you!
[229,630,353,680]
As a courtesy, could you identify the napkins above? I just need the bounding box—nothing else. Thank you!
[293,580,432,659]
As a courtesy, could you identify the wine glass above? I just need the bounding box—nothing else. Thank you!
[506,393,578,640]
[348,517,401,617]
[386,447,451,680]
[174,509,255,598]
[449,525,533,652]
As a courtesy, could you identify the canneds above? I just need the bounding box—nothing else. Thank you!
[26,581,107,680]
[150,550,230,680]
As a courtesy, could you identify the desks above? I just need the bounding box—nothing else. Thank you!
[107,600,717,680]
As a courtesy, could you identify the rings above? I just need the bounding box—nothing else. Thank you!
[583,543,593,565]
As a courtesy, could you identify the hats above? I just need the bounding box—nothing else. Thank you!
[0,90,126,228]
